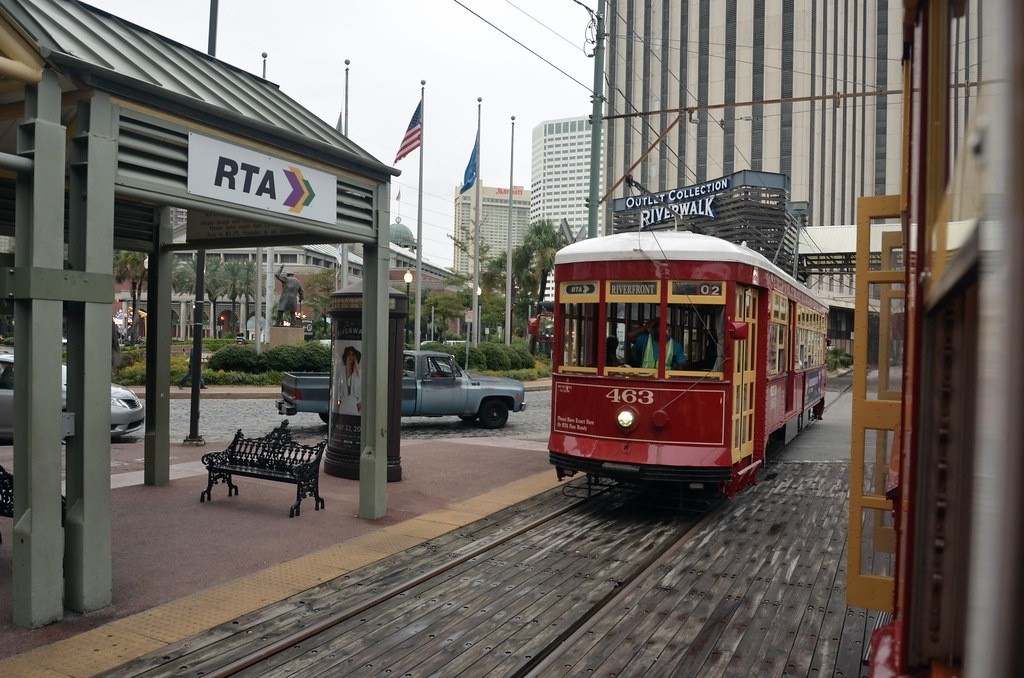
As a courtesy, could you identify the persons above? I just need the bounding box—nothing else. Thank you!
[606,336,622,367]
[0,366,14,389]
[177,342,208,389]
[336,346,361,414]
[274,263,304,326]
[627,318,686,370]
[119,334,144,344]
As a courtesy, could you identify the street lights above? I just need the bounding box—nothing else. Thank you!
[478,286,484,343]
[403,269,413,345]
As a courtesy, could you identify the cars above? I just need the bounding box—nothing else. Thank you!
[0,353,145,443]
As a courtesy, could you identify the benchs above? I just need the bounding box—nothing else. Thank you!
[199,419,329,519]
[0,465,65,545]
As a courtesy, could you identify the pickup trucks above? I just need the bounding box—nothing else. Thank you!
[275,348,528,427]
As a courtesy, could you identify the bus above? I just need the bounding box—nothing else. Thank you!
[548,176,830,512]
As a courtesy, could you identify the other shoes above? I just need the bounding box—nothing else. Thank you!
[201,386,208,389]
[177,384,183,390]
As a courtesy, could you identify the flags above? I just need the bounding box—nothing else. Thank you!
[335,113,343,134]
[393,100,420,166]
[460,132,477,194]
[396,191,401,201]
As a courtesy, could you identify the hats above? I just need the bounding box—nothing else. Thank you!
[343,346,361,365]
